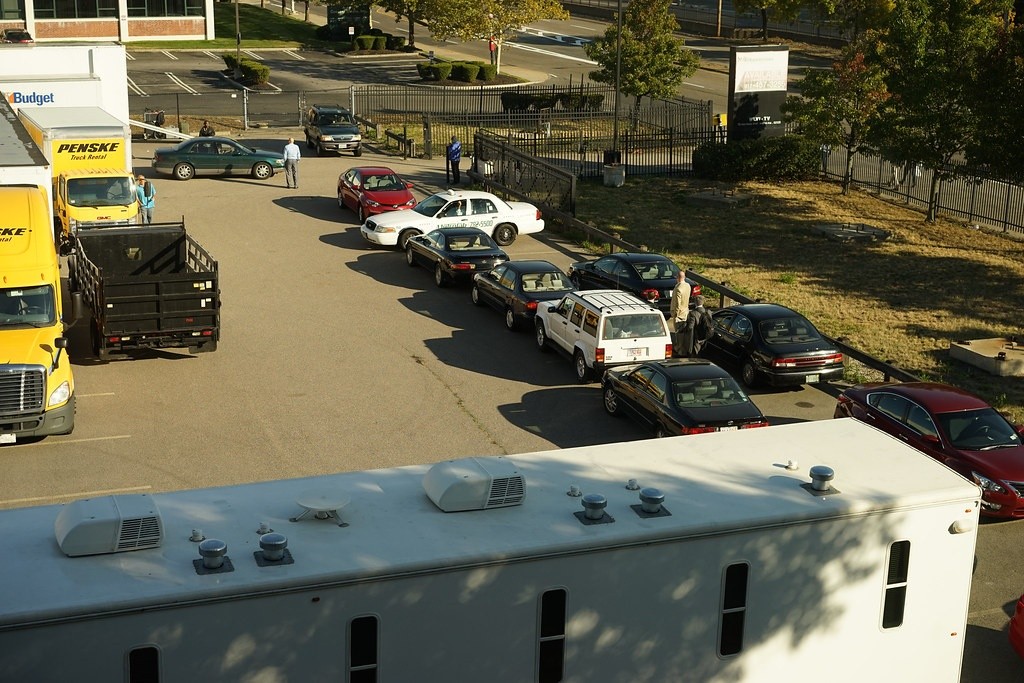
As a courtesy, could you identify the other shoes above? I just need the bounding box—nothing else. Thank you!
[294,186,299,189]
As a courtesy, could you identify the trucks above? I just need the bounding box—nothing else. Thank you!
[0,42,151,253]
[58,214,221,362]
[15,105,148,249]
[0,91,77,449]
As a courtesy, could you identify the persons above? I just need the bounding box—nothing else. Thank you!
[685,294,712,358]
[820,144,831,171]
[199,120,215,137]
[449,136,461,185]
[283,138,301,189]
[888,160,917,191]
[136,175,156,224]
[217,144,224,154]
[670,271,691,353]
[455,201,463,216]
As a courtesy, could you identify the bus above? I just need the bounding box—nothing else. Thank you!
[0,416,983,683]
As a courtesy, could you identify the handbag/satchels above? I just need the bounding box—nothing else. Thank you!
[674,321,687,333]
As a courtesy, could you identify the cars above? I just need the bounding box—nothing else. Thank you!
[834,380,1024,524]
[702,303,845,391]
[152,135,286,179]
[0,28,34,43]
[602,356,768,439]
[566,253,701,320]
[1007,593,1023,667]
[360,190,546,251]
[404,226,510,289]
[471,258,579,331]
[336,166,417,226]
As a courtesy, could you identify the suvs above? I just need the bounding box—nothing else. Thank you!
[304,103,362,157]
[534,289,673,383]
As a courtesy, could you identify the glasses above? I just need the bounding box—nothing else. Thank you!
[139,178,145,181]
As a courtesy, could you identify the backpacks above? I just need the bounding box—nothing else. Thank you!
[695,309,712,340]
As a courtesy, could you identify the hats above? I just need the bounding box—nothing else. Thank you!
[451,136,457,141]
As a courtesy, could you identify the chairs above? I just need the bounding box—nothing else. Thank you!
[719,390,734,399]
[642,271,651,279]
[536,274,552,287]
[791,327,809,338]
[765,330,779,341]
[738,320,751,333]
[773,320,789,335]
[693,381,717,399]
[678,393,695,405]
[664,270,673,277]
[377,176,393,186]
[524,281,536,291]
[548,279,564,291]
[450,244,458,249]
[469,236,481,247]
[104,178,123,200]
